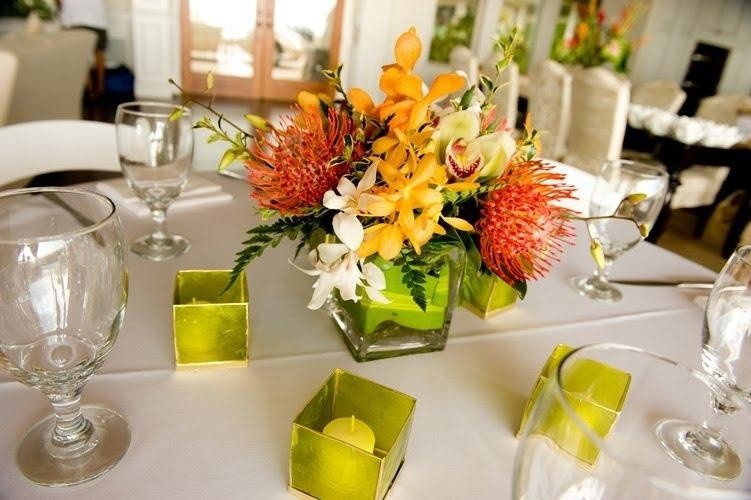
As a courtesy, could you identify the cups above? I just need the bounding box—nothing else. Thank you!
[500,342,751,500]
[456,261,521,320]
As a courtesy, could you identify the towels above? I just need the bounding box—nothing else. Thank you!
[96,168,235,218]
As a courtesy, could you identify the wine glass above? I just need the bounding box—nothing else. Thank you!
[569,160,669,300]
[650,241,751,481]
[115,101,194,261]
[2,187,131,486]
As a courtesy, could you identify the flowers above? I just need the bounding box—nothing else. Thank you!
[548,0,645,76]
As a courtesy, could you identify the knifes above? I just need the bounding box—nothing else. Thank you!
[610,277,721,290]
[43,191,107,247]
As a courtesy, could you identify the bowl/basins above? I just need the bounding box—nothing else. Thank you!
[629,104,743,149]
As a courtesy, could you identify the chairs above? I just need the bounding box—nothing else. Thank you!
[0,116,163,193]
[0,19,105,119]
[163,26,583,312]
